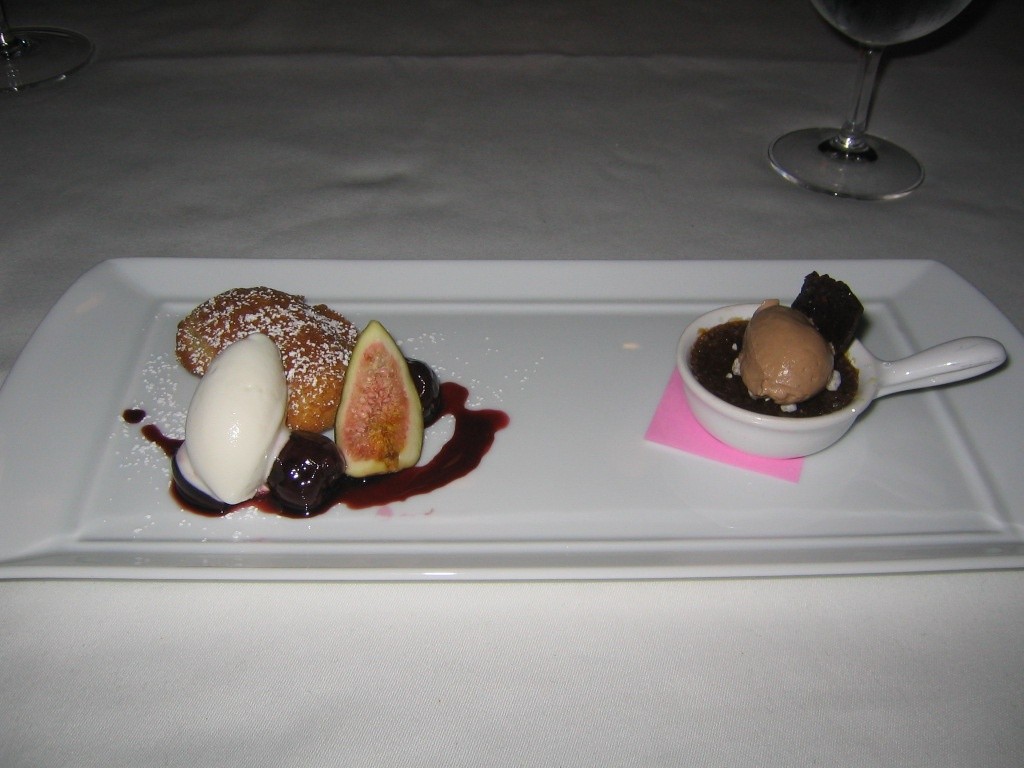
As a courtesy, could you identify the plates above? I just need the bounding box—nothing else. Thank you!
[0,256,1022,582]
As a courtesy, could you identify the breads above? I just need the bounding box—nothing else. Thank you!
[176,287,368,437]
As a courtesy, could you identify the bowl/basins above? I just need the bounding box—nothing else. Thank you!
[678,300,1008,462]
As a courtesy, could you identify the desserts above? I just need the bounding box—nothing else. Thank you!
[693,269,865,418]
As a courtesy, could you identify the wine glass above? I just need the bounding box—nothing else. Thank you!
[764,0,976,201]
[1,12,95,97]
